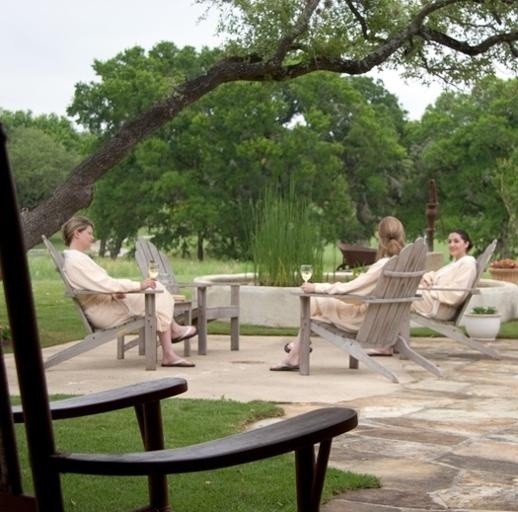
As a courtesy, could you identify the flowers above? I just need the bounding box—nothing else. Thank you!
[490,259,518,269]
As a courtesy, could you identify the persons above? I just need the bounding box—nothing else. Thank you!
[61,216,198,368]
[364,229,477,361]
[270,216,408,372]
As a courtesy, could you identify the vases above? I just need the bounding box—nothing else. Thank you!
[489,267,518,286]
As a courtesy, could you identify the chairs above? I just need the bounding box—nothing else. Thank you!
[0,121,357,511]
[41,234,163,370]
[336,244,377,271]
[289,237,445,383]
[135,235,247,355]
[410,239,502,360]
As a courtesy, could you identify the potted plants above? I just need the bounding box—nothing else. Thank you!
[463,306,501,341]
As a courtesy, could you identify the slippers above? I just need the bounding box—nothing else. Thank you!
[162,358,195,367]
[366,348,392,356]
[270,361,300,371]
[285,343,312,354]
[390,346,398,353]
[172,326,198,344]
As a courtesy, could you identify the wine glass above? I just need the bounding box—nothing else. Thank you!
[148,263,158,279]
[299,264,314,283]
